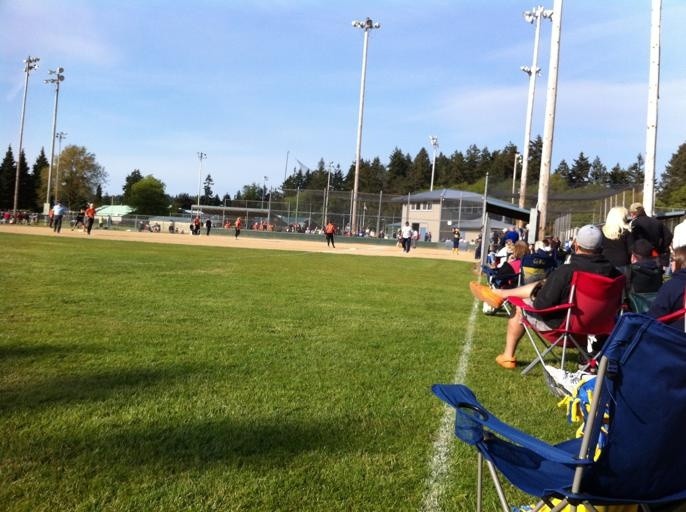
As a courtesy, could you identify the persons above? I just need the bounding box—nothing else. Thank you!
[0,199,98,236]
[451,224,461,256]
[468,201,686,396]
[137,215,432,255]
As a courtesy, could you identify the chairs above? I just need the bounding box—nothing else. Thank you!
[481,252,685,389]
[433,315,686,509]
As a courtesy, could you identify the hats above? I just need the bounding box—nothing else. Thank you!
[629,239,652,256]
[629,203,641,212]
[575,224,603,251]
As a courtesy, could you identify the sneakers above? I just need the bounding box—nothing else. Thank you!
[469,280,504,308]
[543,365,584,397]
[495,354,516,368]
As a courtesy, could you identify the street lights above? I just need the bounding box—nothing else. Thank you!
[42,66,67,217]
[196,152,207,217]
[13,56,40,224]
[324,160,334,227]
[261,176,268,209]
[352,17,380,236]
[429,136,440,192]
[511,5,554,242]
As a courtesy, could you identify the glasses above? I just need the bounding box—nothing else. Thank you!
[670,257,675,262]
